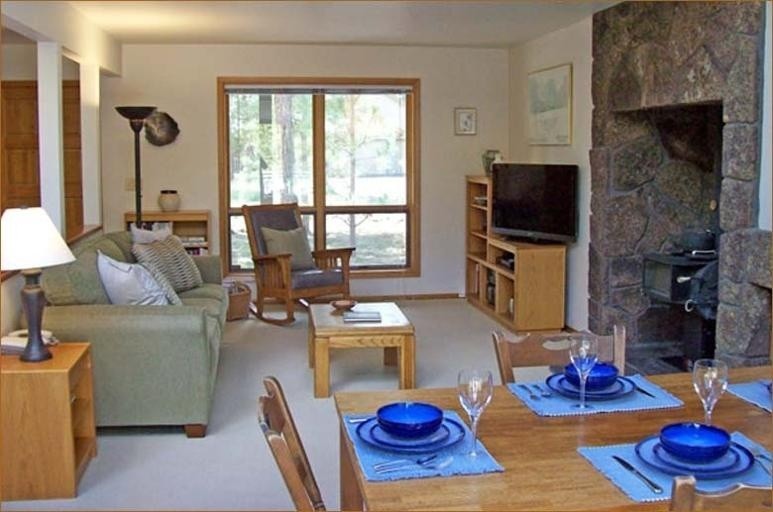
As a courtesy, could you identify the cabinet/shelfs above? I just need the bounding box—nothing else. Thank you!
[125,214,212,254]
[0,344,94,500]
[463,177,568,335]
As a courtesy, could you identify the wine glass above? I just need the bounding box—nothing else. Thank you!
[459,367,493,460]
[693,359,728,421]
[571,335,599,409]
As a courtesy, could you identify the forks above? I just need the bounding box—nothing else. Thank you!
[516,380,542,401]
[750,444,773,467]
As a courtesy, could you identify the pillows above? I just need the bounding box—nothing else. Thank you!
[130,221,174,246]
[259,225,315,271]
[95,251,167,306]
[139,261,183,306]
[132,236,203,293]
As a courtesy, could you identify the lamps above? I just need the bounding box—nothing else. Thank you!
[115,106,158,220]
[1,209,76,361]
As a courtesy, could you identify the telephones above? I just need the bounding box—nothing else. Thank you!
[0,329,58,353]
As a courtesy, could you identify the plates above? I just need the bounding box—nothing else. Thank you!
[634,436,753,479]
[357,417,466,450]
[545,373,635,400]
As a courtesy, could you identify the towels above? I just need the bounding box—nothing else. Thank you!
[575,431,773,504]
[342,407,505,483]
[721,377,773,420]
[507,374,686,415]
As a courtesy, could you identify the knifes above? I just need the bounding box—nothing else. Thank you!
[619,372,654,400]
[611,454,666,494]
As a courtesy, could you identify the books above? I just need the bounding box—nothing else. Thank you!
[343,311,381,323]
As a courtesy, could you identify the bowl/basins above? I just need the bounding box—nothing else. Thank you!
[562,361,619,392]
[330,300,357,311]
[376,403,442,437]
[658,423,729,465]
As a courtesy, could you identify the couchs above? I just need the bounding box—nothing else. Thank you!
[21,232,228,438]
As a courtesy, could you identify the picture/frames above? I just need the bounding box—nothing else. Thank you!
[524,63,574,146]
[453,106,477,137]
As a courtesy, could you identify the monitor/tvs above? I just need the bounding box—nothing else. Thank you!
[491,163,581,246]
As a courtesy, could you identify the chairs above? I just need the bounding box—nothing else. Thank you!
[491,326,626,385]
[257,377,326,512]
[242,202,356,325]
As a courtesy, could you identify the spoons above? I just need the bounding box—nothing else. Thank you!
[374,453,436,470]
[531,383,552,397]
